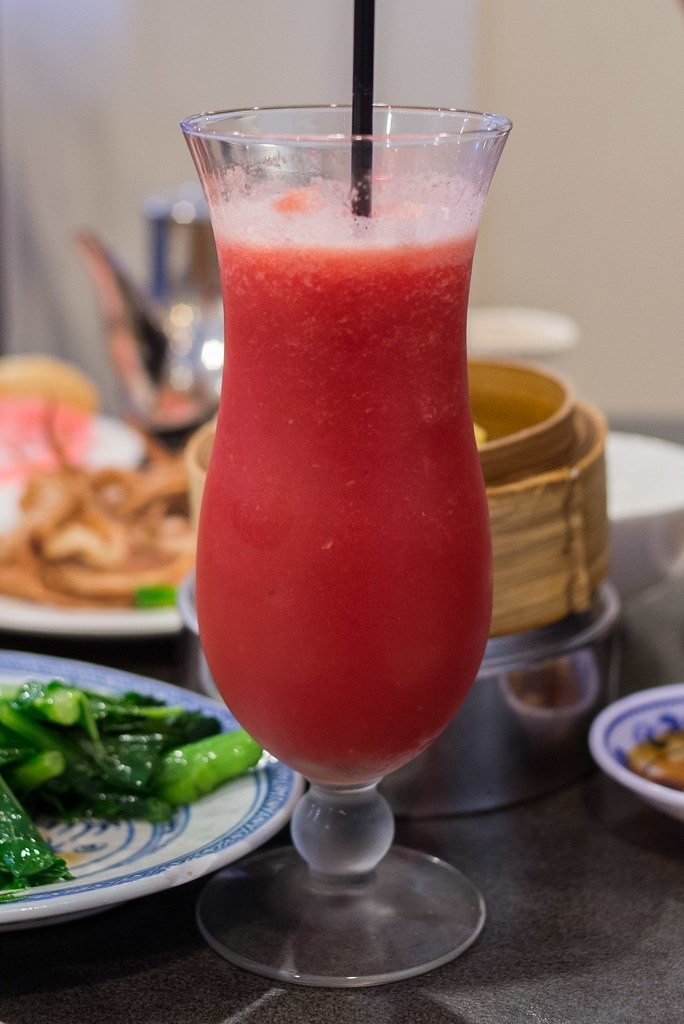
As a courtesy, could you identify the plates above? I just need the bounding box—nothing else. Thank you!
[0,648,305,932]
[0,595,183,636]
[589,683,684,820]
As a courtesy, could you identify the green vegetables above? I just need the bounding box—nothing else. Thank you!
[0,679,262,890]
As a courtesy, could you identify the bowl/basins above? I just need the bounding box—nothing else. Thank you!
[605,430,684,594]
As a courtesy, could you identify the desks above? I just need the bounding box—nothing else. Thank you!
[0,410,684,1024]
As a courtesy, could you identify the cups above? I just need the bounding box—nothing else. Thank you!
[466,307,575,387]
[147,204,225,453]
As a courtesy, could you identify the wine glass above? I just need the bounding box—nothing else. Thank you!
[182,104,513,988]
